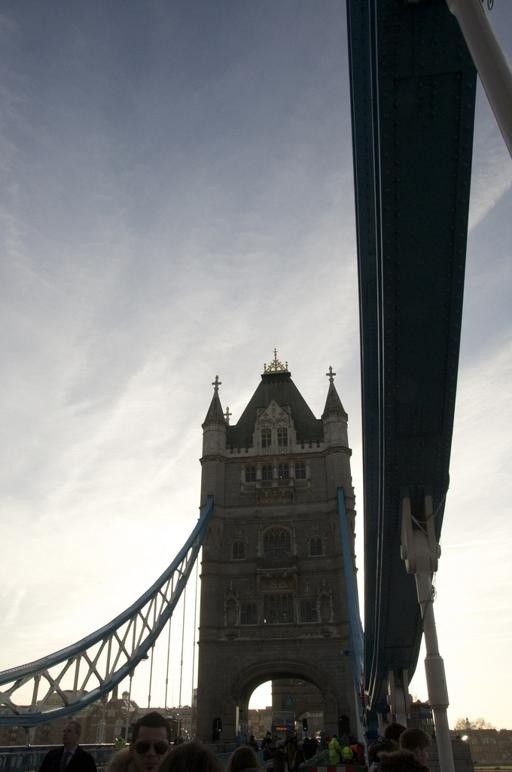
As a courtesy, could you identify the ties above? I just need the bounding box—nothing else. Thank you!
[62,752,72,766]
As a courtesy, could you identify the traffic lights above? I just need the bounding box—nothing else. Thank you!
[217,720,222,731]
[302,718,309,732]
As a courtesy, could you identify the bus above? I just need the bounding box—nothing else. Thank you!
[272,710,295,737]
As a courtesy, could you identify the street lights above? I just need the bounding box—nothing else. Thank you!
[126,649,148,744]
